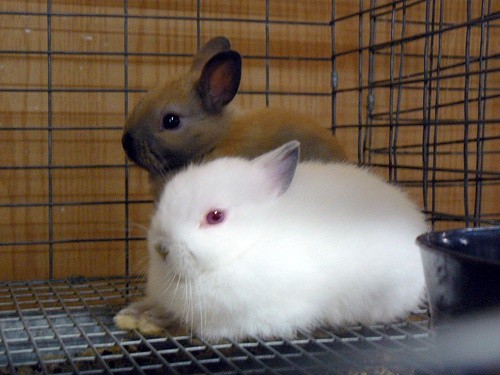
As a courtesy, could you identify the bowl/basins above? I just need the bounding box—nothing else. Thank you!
[417,226,500,334]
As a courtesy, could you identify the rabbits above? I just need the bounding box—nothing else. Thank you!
[121,34,347,210]
[111,138,431,344]
